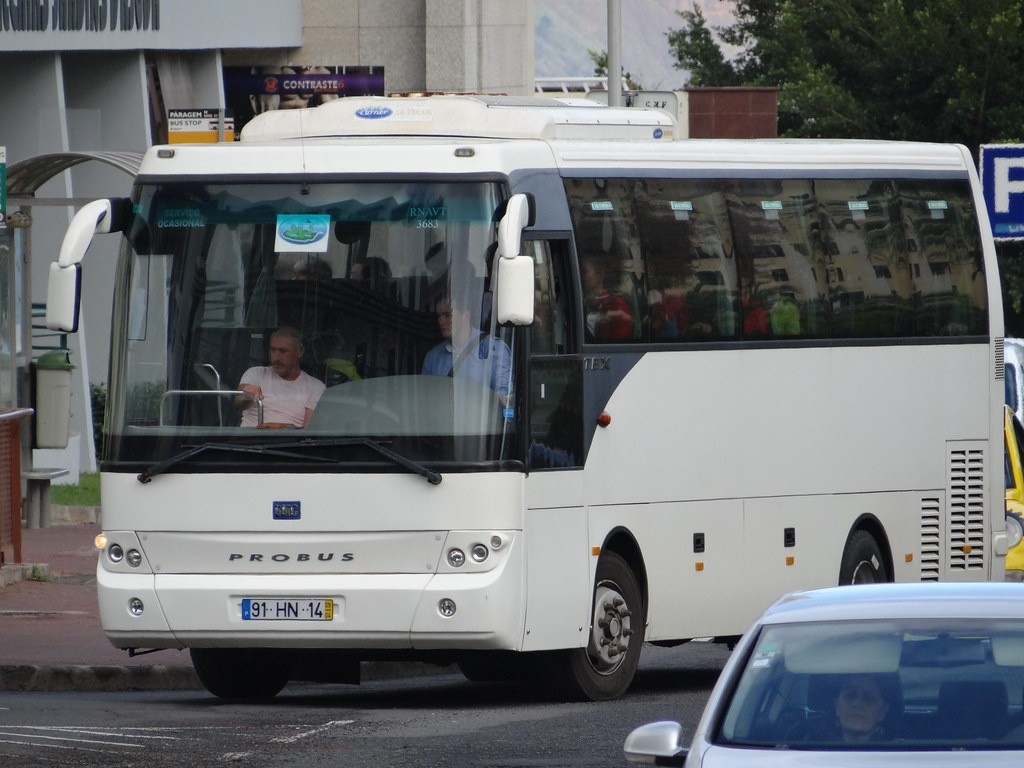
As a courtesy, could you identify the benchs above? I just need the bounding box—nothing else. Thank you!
[20,468,71,531]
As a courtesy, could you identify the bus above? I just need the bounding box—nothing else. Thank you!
[44,92,1007,701]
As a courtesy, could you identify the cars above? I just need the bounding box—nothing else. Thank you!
[1004,404,1024,582]
[623,581,1023,767]
[1003,340,1024,426]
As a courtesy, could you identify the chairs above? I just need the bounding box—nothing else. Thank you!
[784,671,904,743]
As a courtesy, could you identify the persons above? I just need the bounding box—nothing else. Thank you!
[580,249,633,341]
[812,671,901,743]
[236,328,326,429]
[423,290,513,412]
[292,253,979,376]
